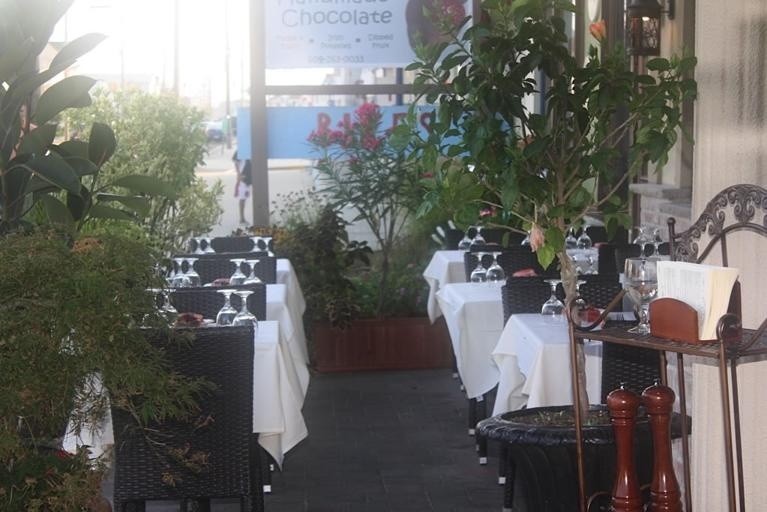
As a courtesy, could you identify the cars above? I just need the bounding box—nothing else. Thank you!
[205,123,223,142]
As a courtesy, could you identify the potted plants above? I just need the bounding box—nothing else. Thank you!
[383,0,700,511]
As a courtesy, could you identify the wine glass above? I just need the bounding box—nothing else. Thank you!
[458,225,504,283]
[632,224,666,257]
[144,237,274,331]
[626,260,659,334]
[543,279,587,324]
[565,223,600,274]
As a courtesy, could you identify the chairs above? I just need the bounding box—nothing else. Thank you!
[445,224,691,512]
[102,234,277,512]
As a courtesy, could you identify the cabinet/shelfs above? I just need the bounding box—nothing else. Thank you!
[563,185,767,511]
